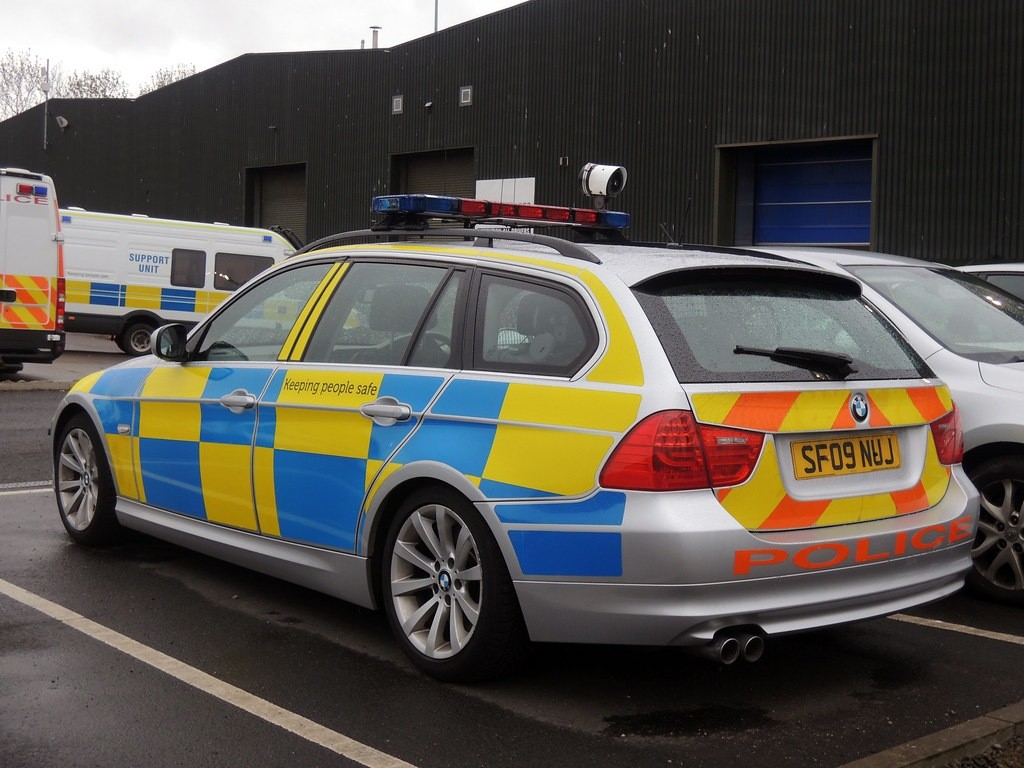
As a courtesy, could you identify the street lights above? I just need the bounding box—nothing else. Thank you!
[38,82,49,166]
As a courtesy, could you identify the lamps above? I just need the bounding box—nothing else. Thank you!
[56,116,70,128]
[269,125,279,132]
[424,101,432,111]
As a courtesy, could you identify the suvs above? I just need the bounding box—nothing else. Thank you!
[46,160,980,683]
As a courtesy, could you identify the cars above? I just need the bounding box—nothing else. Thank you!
[672,242,1024,610]
[862,261,1024,355]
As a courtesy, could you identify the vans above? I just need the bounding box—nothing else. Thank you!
[0,167,67,370]
[59,206,371,357]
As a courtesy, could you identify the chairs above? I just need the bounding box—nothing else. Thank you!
[497,291,581,365]
[347,283,440,367]
[674,314,742,371]
